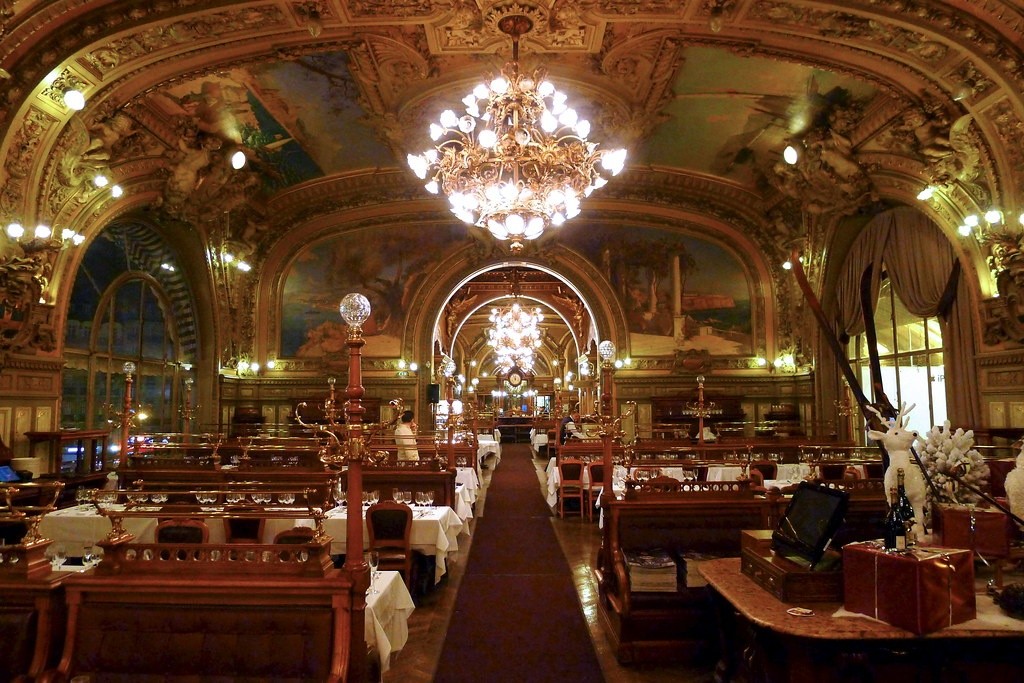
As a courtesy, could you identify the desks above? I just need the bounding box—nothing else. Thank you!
[0,429,1024,683]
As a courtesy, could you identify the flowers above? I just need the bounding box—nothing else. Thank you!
[912,420,990,523]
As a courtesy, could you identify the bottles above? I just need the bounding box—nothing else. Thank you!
[896,469,917,548]
[883,486,907,553]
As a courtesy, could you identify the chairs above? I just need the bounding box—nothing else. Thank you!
[749,459,777,480]
[154,518,210,562]
[820,464,847,481]
[222,500,266,546]
[587,459,603,522]
[554,458,585,520]
[274,526,316,546]
[863,463,886,480]
[366,502,412,591]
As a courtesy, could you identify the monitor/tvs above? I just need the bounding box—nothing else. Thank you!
[0,465,21,483]
[771,481,850,564]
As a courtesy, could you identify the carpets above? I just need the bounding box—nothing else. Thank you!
[432,442,604,683]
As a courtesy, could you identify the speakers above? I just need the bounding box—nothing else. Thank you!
[426,384,439,404]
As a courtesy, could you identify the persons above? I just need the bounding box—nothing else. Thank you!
[560,411,585,446]
[899,108,954,158]
[87,115,144,161]
[394,411,420,462]
[538,406,549,414]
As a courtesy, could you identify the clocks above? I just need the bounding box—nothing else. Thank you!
[507,366,523,387]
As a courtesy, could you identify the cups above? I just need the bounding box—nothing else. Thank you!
[185,453,300,470]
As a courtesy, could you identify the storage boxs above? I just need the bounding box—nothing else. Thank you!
[843,539,977,636]
[742,530,844,605]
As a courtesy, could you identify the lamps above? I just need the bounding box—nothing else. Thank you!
[758,358,772,369]
[239,361,275,374]
[90,174,108,189]
[959,209,1024,279]
[451,362,487,396]
[553,358,631,391]
[224,254,251,273]
[406,15,627,252]
[231,149,246,170]
[61,87,84,112]
[397,360,418,372]
[781,257,793,271]
[773,352,793,367]
[784,142,805,166]
[109,185,123,199]
[7,224,86,246]
[487,268,546,372]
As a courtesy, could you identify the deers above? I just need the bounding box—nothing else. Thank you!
[866,401,926,543]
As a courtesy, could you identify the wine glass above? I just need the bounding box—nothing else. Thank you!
[421,456,469,473]
[44,540,102,573]
[567,451,700,489]
[194,483,295,509]
[76,487,168,517]
[335,487,435,516]
